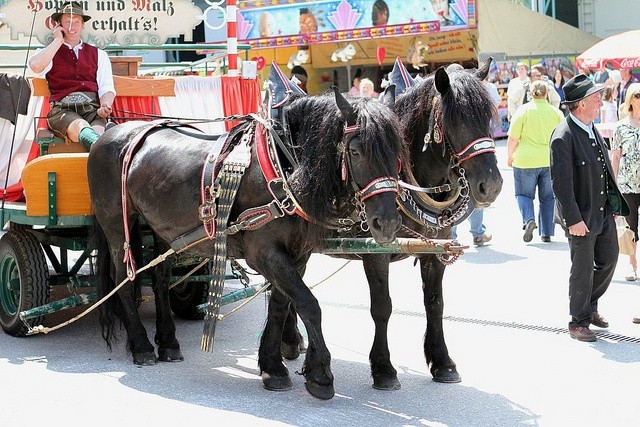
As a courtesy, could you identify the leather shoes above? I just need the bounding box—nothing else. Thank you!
[569,326,597,342]
[590,312,609,328]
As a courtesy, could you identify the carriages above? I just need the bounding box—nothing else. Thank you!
[0,0,503,399]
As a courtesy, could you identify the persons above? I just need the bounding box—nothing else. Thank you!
[508,80,565,243]
[347,77,360,101]
[616,67,638,105]
[550,74,631,342]
[552,68,567,118]
[599,84,617,125]
[507,64,531,119]
[29,1,116,153]
[611,82,639,282]
[447,64,491,246]
[527,66,561,110]
[358,78,376,100]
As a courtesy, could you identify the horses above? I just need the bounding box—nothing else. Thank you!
[277,57,503,390]
[85,84,405,400]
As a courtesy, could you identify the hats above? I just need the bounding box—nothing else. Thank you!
[50,2,92,22]
[561,74,605,105]
[620,83,640,114]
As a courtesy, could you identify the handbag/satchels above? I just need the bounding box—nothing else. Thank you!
[613,216,636,256]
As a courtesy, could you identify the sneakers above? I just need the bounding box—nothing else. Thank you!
[541,236,551,242]
[473,233,492,246]
[523,220,536,242]
